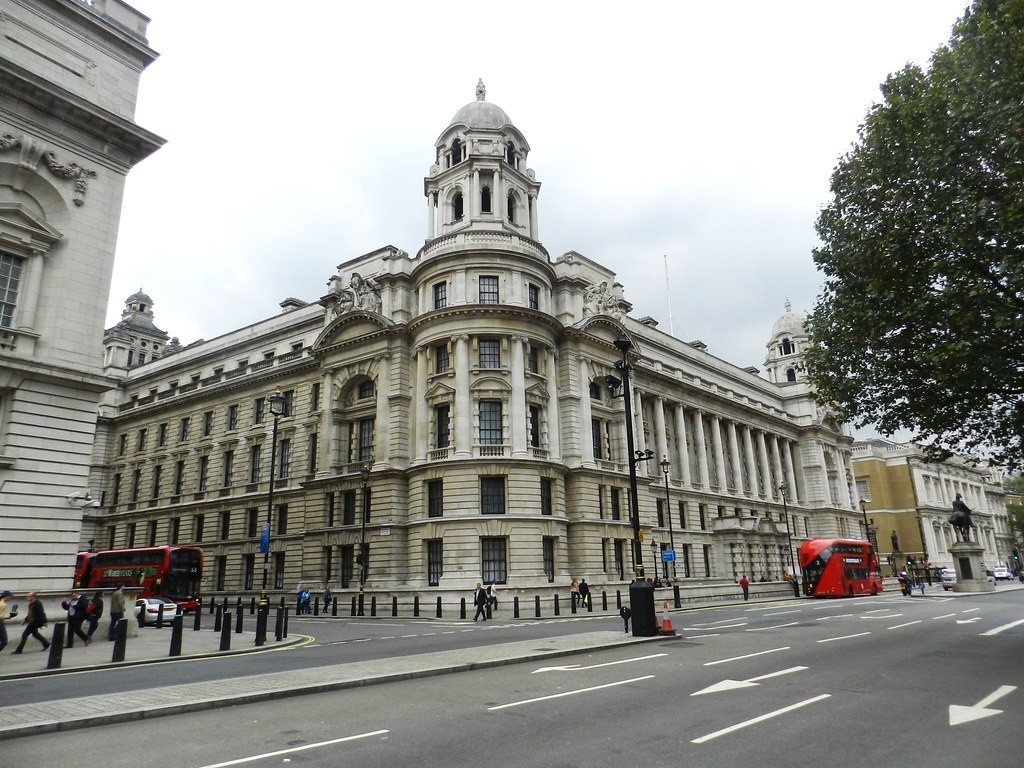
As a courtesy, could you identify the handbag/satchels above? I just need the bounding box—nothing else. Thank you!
[305,599,311,604]
[584,594,588,605]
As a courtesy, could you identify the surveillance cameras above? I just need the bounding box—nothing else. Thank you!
[66,493,79,499]
[93,502,101,509]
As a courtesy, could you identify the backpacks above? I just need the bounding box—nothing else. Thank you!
[87,601,95,613]
[487,586,491,594]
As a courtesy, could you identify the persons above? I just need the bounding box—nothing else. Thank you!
[760,576,768,582]
[299,585,313,614]
[740,575,749,601]
[579,579,589,608]
[87,591,104,640]
[12,591,50,654]
[952,493,974,528]
[647,577,672,588]
[571,578,580,607]
[473,583,487,621]
[0,591,17,651]
[880,569,944,591]
[322,587,332,613]
[490,580,500,611]
[109,584,126,640]
[776,571,792,581]
[62,591,90,648]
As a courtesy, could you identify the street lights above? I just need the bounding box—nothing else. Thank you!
[612,338,655,636]
[358,464,371,615]
[779,484,800,597]
[254,392,286,647]
[859,499,873,543]
[659,458,682,608]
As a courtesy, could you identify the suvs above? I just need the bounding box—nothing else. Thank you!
[942,571,957,591]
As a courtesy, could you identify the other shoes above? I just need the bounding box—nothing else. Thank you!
[482,619,486,621]
[63,645,73,648]
[42,643,51,651]
[308,611,312,614]
[85,638,90,646]
[108,635,116,641]
[12,651,22,654]
[473,618,477,621]
[323,609,328,613]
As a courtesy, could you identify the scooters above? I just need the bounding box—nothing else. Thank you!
[898,577,913,596]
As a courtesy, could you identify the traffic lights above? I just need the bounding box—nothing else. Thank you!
[887,556,890,563]
[907,556,911,565]
[918,557,923,564]
[1012,549,1019,560]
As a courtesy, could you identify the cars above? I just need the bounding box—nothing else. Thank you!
[1019,567,1024,584]
[994,567,1014,580]
[135,597,177,628]
[983,570,996,586]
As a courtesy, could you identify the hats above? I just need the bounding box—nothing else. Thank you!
[493,579,497,583]
[2,591,13,596]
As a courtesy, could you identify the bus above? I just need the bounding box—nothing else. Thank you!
[799,538,883,597]
[73,553,95,590]
[88,546,203,612]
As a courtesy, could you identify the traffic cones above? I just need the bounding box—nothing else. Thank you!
[656,602,678,636]
